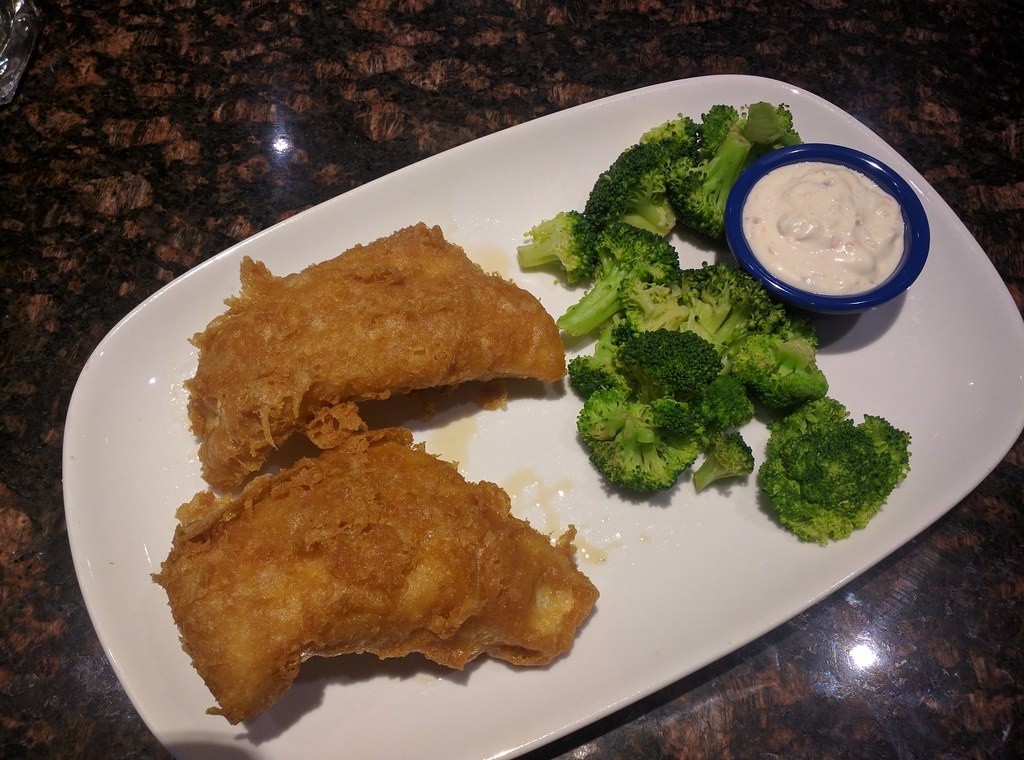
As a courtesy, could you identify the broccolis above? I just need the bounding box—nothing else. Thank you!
[514,102,913,546]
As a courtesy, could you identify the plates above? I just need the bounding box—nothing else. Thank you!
[61,75,1024,760]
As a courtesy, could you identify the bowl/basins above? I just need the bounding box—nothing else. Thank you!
[723,141,934,316]
